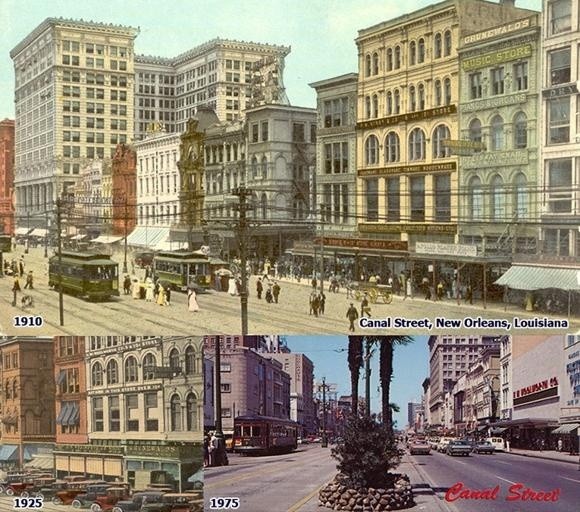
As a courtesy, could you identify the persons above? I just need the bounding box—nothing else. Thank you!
[1,233,580,319]
[558,436,563,452]
[347,303,359,331]
[203,435,211,467]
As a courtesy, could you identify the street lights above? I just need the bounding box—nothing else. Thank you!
[318,376,328,447]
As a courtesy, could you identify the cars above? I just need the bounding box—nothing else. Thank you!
[296,435,344,444]
[0,468,203,512]
[348,276,395,304]
[394,432,506,457]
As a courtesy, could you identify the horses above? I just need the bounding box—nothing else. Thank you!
[340,275,360,299]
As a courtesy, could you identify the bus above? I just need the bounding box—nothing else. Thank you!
[0,233,12,254]
[47,247,122,300]
[151,249,211,293]
[233,412,299,455]
